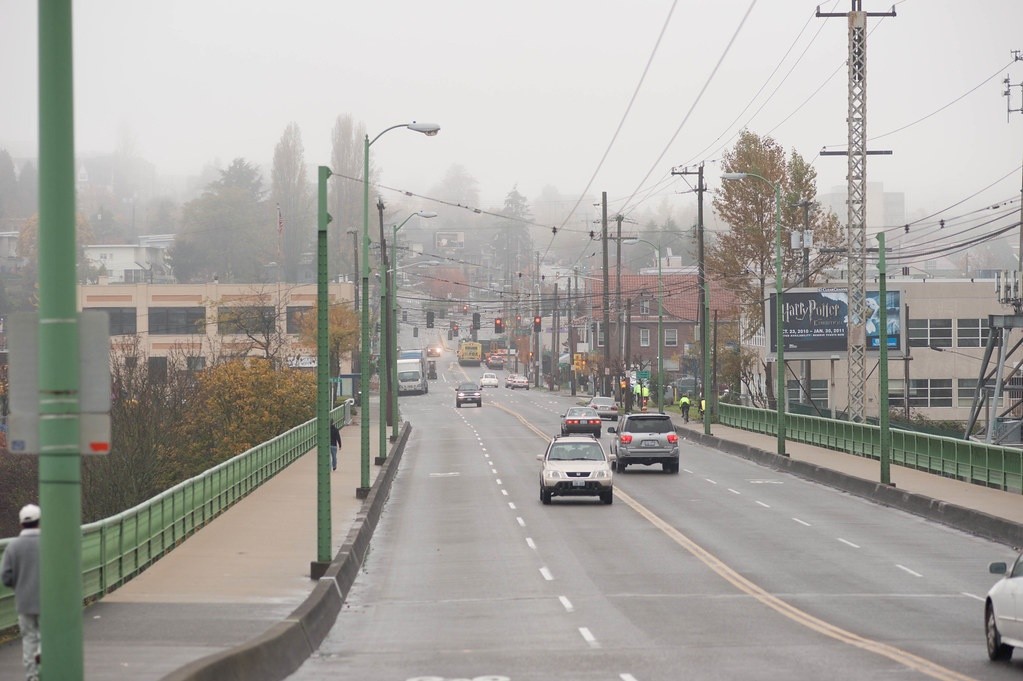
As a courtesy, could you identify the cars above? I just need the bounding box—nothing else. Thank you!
[505,374,517,388]
[512,375,529,390]
[585,396,620,421]
[427,343,441,357]
[480,372,499,388]
[484,352,504,370]
[561,407,601,437]
[984,550,1023,662]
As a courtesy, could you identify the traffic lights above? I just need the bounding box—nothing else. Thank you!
[534,315,542,332]
[440,308,445,319]
[413,327,419,338]
[583,353,588,366]
[516,314,522,327]
[375,321,382,332]
[453,325,459,337]
[450,321,456,329]
[426,311,435,328]
[473,313,481,329]
[402,310,408,321]
[447,329,453,341]
[600,321,606,333]
[495,318,504,334]
[463,305,468,315]
[591,321,596,333]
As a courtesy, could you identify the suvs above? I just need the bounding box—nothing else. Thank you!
[454,382,483,408]
[536,433,616,505]
[605,412,681,475]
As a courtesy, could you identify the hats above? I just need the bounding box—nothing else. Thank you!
[19,504,40,522]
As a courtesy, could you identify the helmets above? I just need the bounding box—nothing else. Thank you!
[702,398,705,400]
[682,394,687,397]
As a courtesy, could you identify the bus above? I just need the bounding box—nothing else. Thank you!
[457,342,482,367]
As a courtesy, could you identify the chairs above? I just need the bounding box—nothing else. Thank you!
[552,448,567,458]
[629,423,638,433]
[582,447,595,459]
[654,423,667,432]
[585,411,593,416]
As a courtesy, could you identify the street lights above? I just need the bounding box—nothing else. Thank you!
[621,237,667,415]
[512,271,543,389]
[388,210,439,441]
[374,197,442,468]
[719,172,791,457]
[357,119,441,502]
[504,284,533,383]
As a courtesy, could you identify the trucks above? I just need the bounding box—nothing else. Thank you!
[396,358,429,395]
[399,348,428,381]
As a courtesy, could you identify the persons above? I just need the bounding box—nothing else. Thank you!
[630,383,650,406]
[698,398,706,419]
[621,379,626,395]
[0,504,42,681]
[330,418,341,470]
[679,394,691,422]
